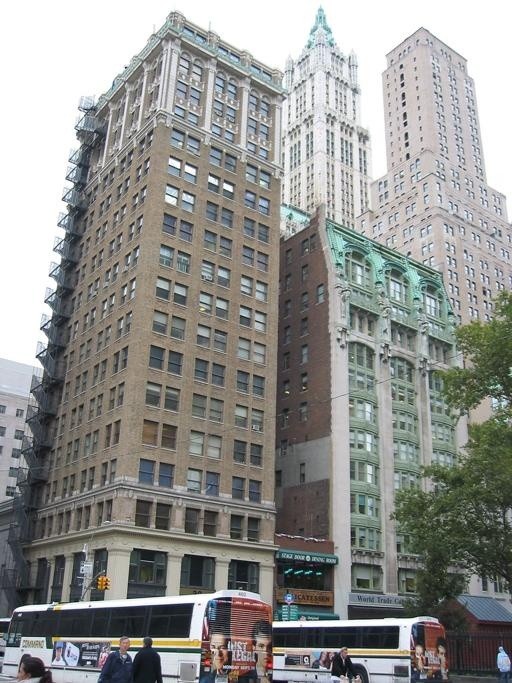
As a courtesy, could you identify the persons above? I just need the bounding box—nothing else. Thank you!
[98,644,110,667]
[97,637,134,682]
[312,652,336,670]
[330,647,360,683]
[16,657,53,682]
[496,646,512,683]
[51,641,66,665]
[132,637,163,682]
[198,617,237,683]
[412,636,450,681]
[232,619,273,683]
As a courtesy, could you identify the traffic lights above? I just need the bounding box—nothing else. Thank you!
[97,576,111,592]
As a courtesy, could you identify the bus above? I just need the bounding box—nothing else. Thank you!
[274,616,448,682]
[0,589,272,683]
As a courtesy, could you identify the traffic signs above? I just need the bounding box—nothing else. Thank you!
[282,605,298,620]
